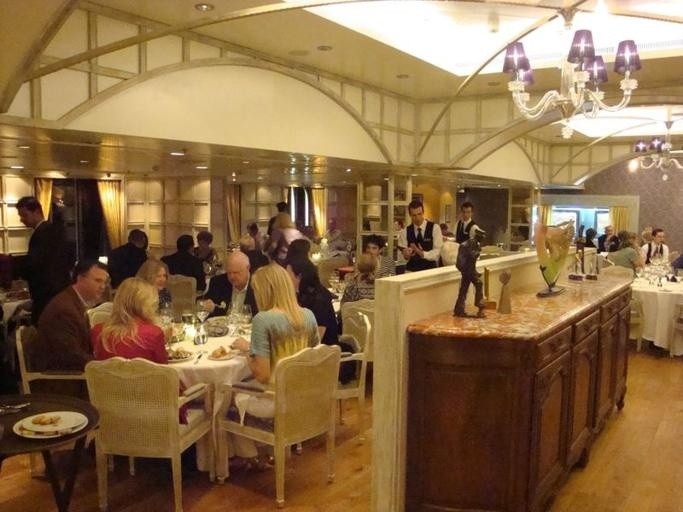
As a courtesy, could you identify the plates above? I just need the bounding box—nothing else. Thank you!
[210,353,233,362]
[10,409,87,439]
[168,356,193,362]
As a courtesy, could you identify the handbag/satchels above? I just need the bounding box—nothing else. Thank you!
[337,341,357,384]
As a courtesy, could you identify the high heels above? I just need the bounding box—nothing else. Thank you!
[250,453,277,474]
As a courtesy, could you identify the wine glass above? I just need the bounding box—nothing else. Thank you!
[194,326,208,355]
[158,300,172,325]
[171,316,183,348]
[239,305,251,324]
[195,299,209,324]
[327,270,353,292]
[635,253,670,290]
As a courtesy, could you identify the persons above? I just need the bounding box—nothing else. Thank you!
[454,228,486,314]
[394,219,403,232]
[365,234,395,279]
[90,277,189,425]
[106,199,343,344]
[440,222,449,240]
[443,201,482,262]
[340,252,380,305]
[30,259,111,371]
[231,264,321,472]
[642,228,669,265]
[397,200,444,273]
[582,224,644,276]
[10,195,71,327]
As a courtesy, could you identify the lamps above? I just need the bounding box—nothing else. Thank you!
[635,105,683,181]
[503,8,641,140]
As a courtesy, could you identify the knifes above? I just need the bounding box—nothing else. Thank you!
[193,353,203,365]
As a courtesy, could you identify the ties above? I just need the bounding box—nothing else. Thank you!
[416,228,423,241]
[460,222,464,236]
[654,247,659,258]
